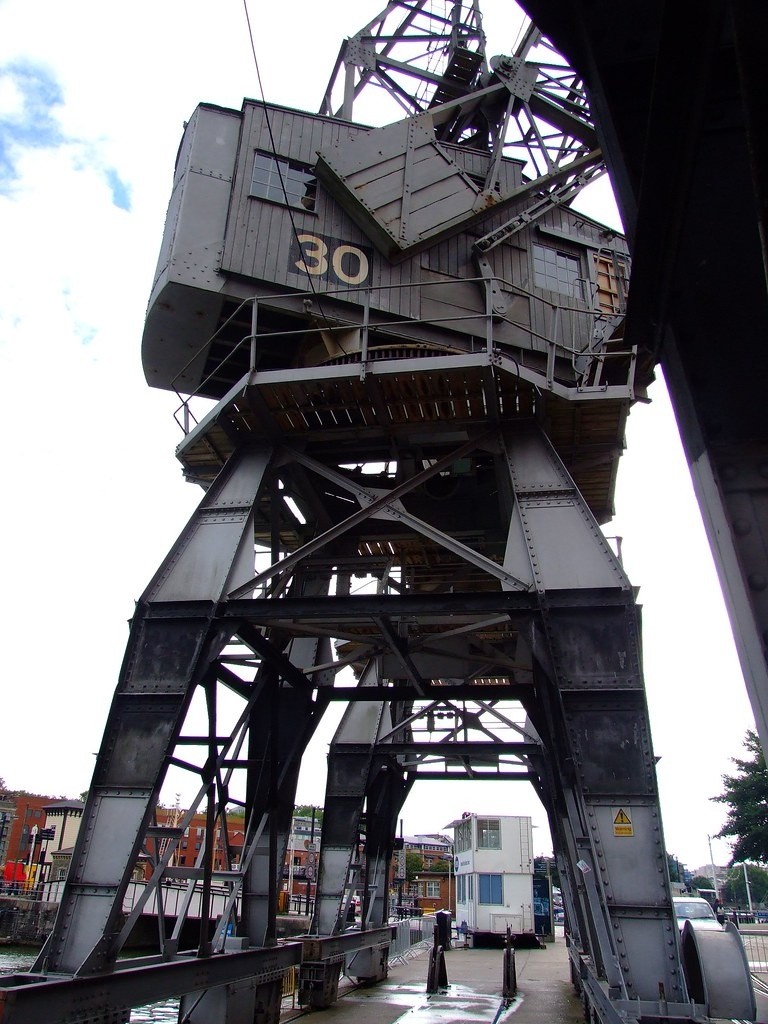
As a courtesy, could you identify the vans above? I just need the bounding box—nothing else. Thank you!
[672,896,724,933]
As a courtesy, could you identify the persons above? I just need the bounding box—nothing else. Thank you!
[166,879,171,886]
[715,904,724,925]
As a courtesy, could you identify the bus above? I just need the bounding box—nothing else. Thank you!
[698,889,723,909]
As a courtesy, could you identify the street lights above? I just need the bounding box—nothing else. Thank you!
[677,864,687,895]
[305,807,324,915]
